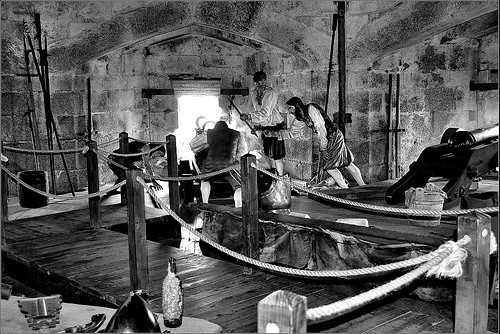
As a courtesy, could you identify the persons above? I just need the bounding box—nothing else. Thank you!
[107,140,165,194]
[240,71,286,159]
[264,96,365,189]
